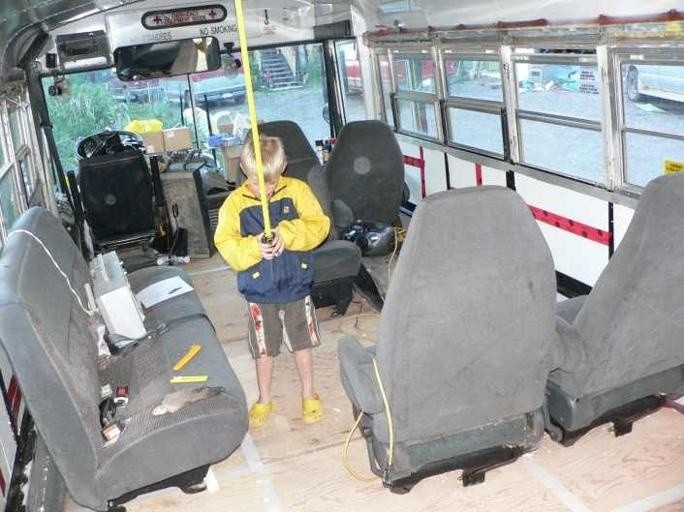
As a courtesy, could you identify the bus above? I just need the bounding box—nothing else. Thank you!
[343,42,458,96]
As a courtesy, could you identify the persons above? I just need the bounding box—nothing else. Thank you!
[213,135,330,430]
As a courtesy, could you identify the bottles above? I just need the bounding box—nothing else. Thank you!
[314,138,336,165]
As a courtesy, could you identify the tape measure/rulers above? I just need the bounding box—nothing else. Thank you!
[235,0,276,247]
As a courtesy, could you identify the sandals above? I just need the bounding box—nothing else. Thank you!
[302,392,324,425]
[248,399,274,428]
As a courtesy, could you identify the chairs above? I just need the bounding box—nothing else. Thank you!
[66,152,164,256]
[236,121,409,314]
[543,173,683,487]
[340,186,557,486]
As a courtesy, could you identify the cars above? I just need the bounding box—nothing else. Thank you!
[625,64,684,103]
[106,53,247,114]
